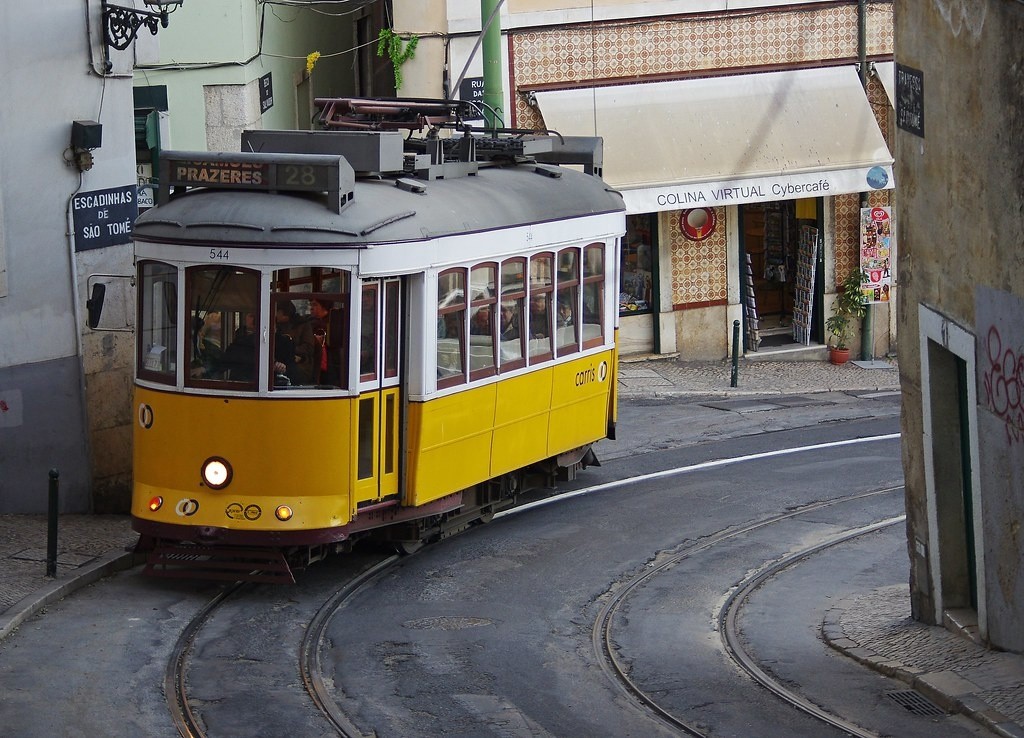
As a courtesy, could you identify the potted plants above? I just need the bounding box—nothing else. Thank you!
[825,266,870,365]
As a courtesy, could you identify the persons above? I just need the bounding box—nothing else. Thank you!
[636,236,653,273]
[444,292,574,342]
[275,297,344,386]
[190,308,299,385]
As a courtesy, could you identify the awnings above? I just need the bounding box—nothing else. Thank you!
[531,64,896,216]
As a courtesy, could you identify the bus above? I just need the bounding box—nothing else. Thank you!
[83,106,625,578]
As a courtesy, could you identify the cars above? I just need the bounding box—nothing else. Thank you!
[437,275,602,379]
